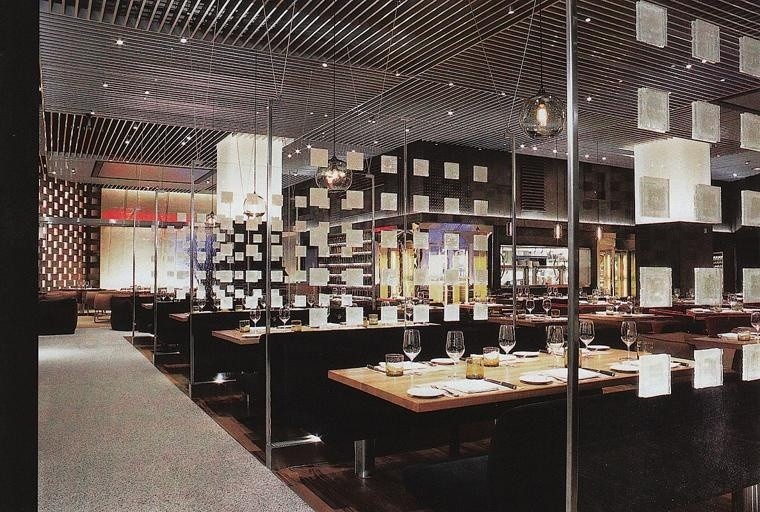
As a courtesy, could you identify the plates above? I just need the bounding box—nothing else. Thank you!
[429,357,455,364]
[609,364,638,372]
[512,350,540,357]
[519,374,552,385]
[405,387,445,399]
[517,260,539,268]
[589,344,610,351]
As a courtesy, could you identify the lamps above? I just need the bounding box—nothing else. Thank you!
[553,136,563,240]
[518,1,566,140]
[315,0,354,195]
[241,42,266,218]
[202,169,221,228]
[596,141,604,241]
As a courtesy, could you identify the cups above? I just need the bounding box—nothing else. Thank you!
[385,352,403,377]
[465,356,484,380]
[637,339,654,356]
[482,346,500,367]
[363,317,368,329]
[332,287,346,296]
[369,314,378,326]
[77,280,90,287]
[563,349,582,369]
[417,291,424,301]
[517,310,526,320]
[468,298,475,305]
[489,298,496,305]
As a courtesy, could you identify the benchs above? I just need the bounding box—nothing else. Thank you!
[81,288,760,512]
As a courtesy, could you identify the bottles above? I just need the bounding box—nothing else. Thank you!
[713,255,723,267]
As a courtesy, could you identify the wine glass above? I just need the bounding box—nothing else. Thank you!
[579,319,595,360]
[521,286,636,317]
[474,289,491,305]
[545,325,565,368]
[619,319,638,362]
[498,324,516,369]
[445,330,466,378]
[405,300,415,326]
[159,289,331,334]
[672,287,760,343]
[402,330,422,377]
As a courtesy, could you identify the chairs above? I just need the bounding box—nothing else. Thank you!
[38,291,78,336]
